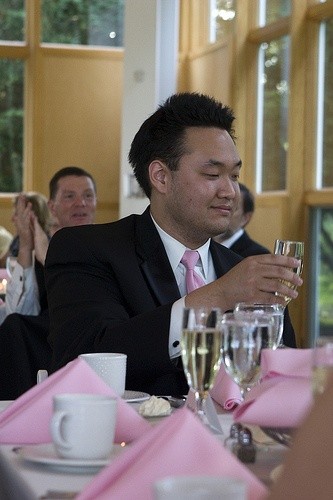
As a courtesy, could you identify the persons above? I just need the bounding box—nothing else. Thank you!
[0,166,98,401]
[42,91,304,401]
[215,182,299,349]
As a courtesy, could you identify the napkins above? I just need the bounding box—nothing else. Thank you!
[210,362,244,410]
[0,357,151,447]
[232,346,333,426]
[74,407,270,500]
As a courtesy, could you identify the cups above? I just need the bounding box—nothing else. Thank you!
[233,304,285,367]
[49,393,118,461]
[80,352,127,397]
[154,474,248,499]
[271,239,306,314]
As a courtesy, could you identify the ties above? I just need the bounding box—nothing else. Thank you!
[180,251,207,295]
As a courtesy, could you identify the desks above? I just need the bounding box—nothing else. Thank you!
[0,340,333,500]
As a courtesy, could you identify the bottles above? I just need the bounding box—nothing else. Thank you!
[223,422,256,465]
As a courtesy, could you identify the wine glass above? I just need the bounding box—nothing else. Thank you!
[179,306,224,437]
[221,313,277,403]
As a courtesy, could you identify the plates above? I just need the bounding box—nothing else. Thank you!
[122,390,154,402]
[19,442,126,474]
